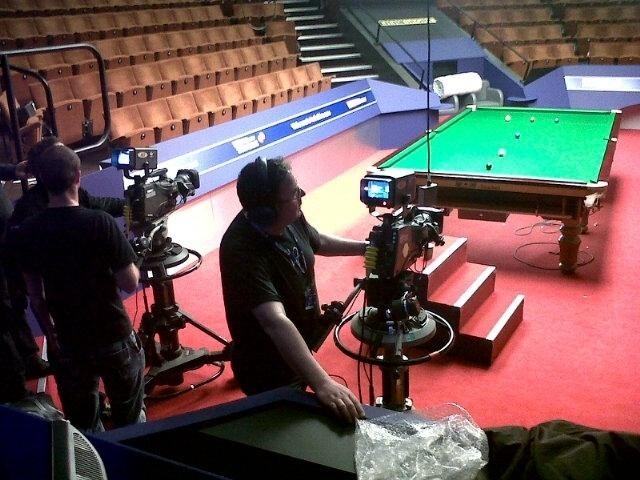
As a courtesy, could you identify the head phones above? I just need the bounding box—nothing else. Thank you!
[248,156,279,226]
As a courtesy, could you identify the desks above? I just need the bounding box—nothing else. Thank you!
[366,104,623,276]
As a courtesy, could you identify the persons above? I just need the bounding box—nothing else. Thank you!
[0,156,55,405]
[5,135,132,421]
[218,155,371,427]
[15,141,148,439]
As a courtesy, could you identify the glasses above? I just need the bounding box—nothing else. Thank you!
[277,177,302,209]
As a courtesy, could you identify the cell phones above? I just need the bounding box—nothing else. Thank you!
[23,99,37,118]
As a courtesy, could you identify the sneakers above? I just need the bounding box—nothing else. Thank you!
[25,353,54,380]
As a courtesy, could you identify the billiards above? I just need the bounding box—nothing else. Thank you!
[515,132,520,138]
[486,163,492,169]
[554,117,559,122]
[530,117,535,122]
[505,116,511,121]
[498,150,504,156]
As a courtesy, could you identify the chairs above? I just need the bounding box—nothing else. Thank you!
[0,0,332,151]
[433,0,640,80]
